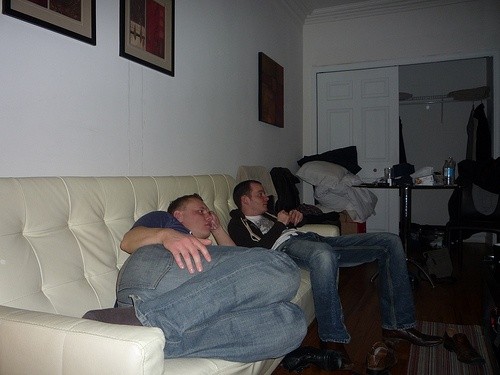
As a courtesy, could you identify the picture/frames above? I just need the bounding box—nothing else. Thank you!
[2,0,96,46]
[258,52,284,129]
[119,0,175,77]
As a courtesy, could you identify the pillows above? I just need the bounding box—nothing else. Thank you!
[296,161,377,220]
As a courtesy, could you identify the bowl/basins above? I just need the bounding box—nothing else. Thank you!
[433,174,443,185]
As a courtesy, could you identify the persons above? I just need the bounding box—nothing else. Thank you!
[228,181,444,362]
[82,195,308,363]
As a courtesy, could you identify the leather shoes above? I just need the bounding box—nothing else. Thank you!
[282,346,345,375]
[366,342,397,373]
[442,329,485,365]
[319,338,355,371]
[382,327,442,346]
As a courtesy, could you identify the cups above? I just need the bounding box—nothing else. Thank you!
[385,168,394,182]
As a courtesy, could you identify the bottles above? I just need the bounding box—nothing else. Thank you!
[388,174,393,186]
[443,157,456,186]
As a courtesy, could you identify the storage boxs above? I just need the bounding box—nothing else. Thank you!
[341,222,366,234]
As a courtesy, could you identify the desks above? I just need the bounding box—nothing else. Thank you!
[353,184,459,258]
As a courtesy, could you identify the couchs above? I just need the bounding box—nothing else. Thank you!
[0,174,340,375]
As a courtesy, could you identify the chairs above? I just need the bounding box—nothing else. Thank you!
[442,157,500,244]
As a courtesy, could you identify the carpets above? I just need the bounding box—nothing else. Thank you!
[405,319,494,375]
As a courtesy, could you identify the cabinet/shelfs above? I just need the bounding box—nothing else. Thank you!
[310,51,500,284]
